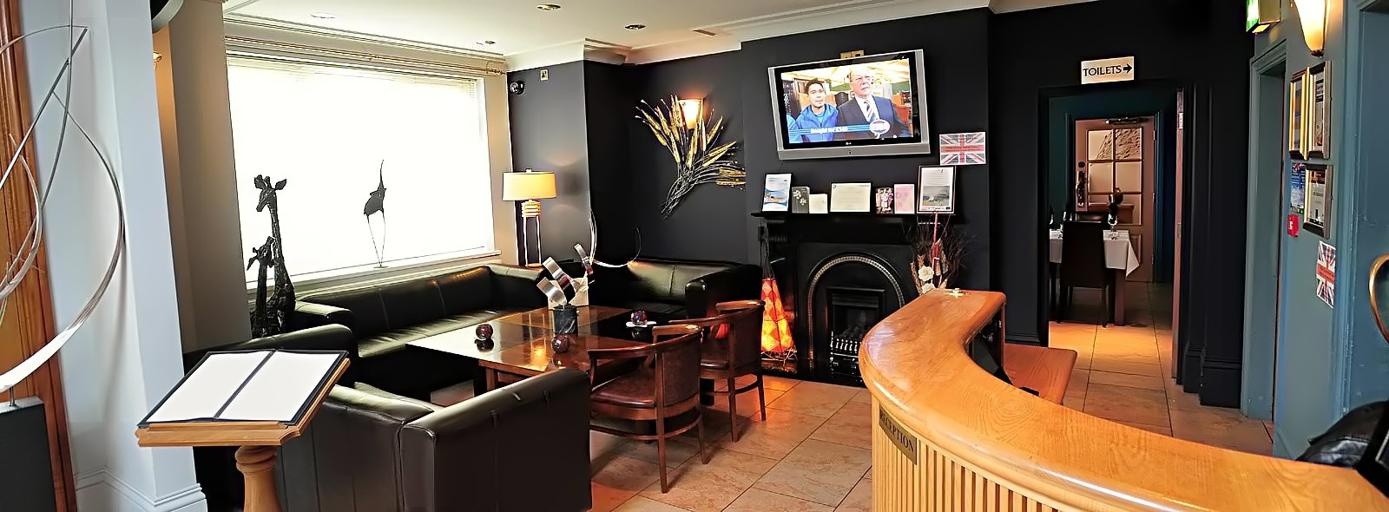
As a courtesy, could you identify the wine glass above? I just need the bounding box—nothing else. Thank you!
[1059,211,1076,237]
[1107,213,1118,236]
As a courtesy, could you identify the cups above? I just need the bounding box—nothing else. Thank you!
[630,309,647,326]
[475,324,494,341]
[551,333,571,352]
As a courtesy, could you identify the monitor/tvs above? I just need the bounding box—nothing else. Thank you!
[767,49,931,161]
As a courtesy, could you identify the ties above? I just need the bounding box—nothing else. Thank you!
[862,100,877,124]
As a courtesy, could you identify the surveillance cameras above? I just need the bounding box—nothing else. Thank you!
[509,81,524,95]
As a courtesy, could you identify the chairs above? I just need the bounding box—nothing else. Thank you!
[1060,222,1115,323]
[586,320,710,494]
[669,300,766,442]
[187,325,593,511]
[565,253,763,329]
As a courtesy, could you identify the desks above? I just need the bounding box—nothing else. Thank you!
[1050,228,1140,327]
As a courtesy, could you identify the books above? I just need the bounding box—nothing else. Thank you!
[761,173,915,214]
[137,348,349,425]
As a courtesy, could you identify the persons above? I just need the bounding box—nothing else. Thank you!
[795,77,838,142]
[832,67,912,141]
[786,115,803,143]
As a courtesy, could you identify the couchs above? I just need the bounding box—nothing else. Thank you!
[294,262,546,392]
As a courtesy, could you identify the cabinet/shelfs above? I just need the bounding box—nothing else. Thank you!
[558,261,581,277]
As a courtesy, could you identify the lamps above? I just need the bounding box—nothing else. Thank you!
[678,98,704,132]
[501,167,557,266]
[1291,1,1324,58]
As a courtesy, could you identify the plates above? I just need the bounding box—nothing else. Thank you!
[625,320,657,329]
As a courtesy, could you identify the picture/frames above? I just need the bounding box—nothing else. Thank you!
[1305,61,1331,161]
[1288,69,1306,161]
[762,165,958,214]
[1302,163,1331,240]
